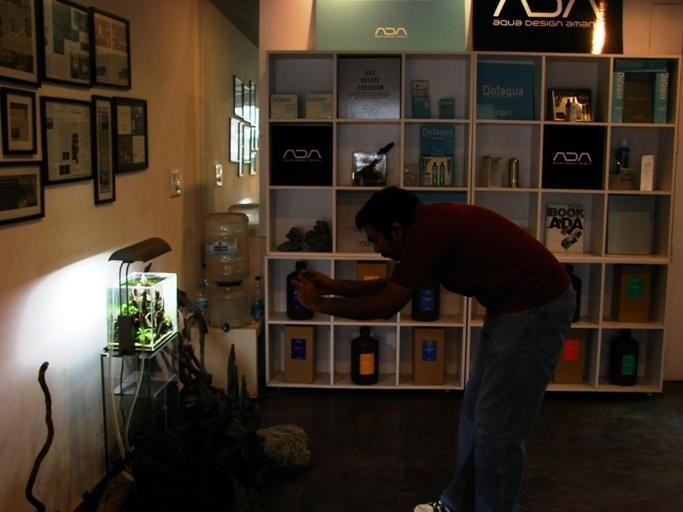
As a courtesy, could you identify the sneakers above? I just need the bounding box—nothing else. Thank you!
[414,499,448,512]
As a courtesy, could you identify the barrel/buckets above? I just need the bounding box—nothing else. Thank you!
[412,285,440,321]
[564,263,581,323]
[204,212,249,283]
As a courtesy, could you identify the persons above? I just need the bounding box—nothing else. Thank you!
[294,184,576,512]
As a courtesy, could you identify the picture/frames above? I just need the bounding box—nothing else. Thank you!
[0,0,148,225]
[228,75,259,178]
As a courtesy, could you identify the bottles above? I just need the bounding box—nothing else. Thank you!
[411,274,439,321]
[192,213,262,327]
[615,136,633,175]
[404,167,412,185]
[564,95,583,122]
[481,154,519,189]
[429,162,447,186]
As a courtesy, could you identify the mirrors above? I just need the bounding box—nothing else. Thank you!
[193,0,265,316]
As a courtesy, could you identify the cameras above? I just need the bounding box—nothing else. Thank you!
[295,273,316,287]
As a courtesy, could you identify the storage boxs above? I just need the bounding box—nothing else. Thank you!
[553,332,586,384]
[284,326,315,383]
[413,328,445,384]
[357,263,388,280]
[611,272,651,321]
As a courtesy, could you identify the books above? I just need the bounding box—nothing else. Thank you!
[615,59,669,122]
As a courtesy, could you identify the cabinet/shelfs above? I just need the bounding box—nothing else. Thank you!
[100,331,180,482]
[268,51,676,394]
